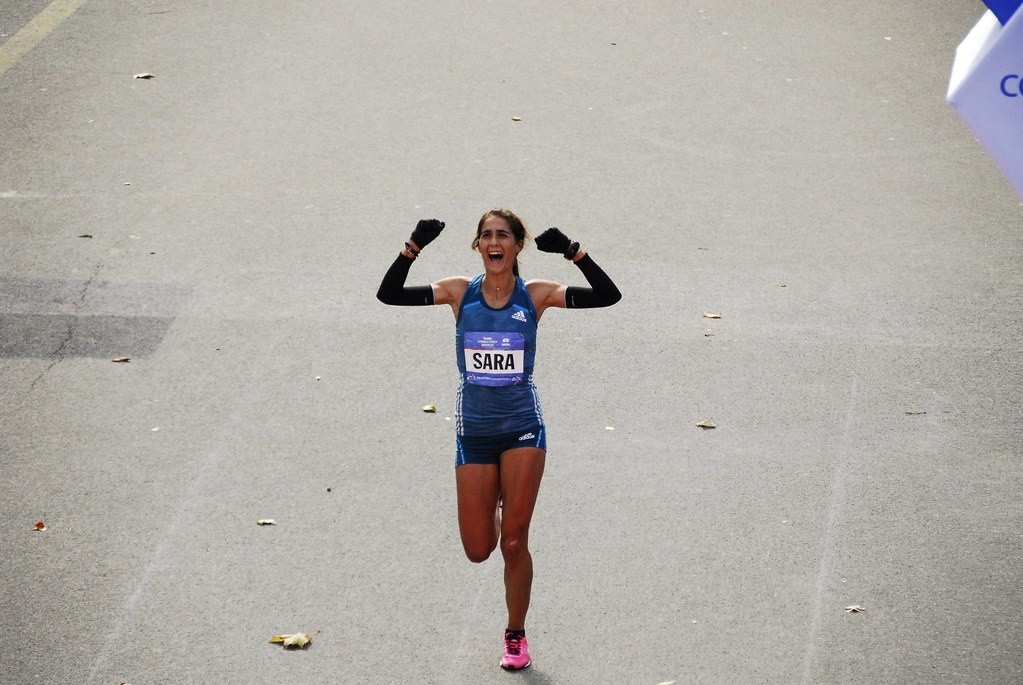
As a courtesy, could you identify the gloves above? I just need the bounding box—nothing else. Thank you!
[409,219,445,249]
[534,227,571,254]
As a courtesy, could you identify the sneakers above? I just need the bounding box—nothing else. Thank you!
[500,628,531,671]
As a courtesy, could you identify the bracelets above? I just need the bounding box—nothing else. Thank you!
[404,242,420,258]
[564,241,580,261]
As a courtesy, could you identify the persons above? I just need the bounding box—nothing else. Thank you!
[377,208,621,672]
